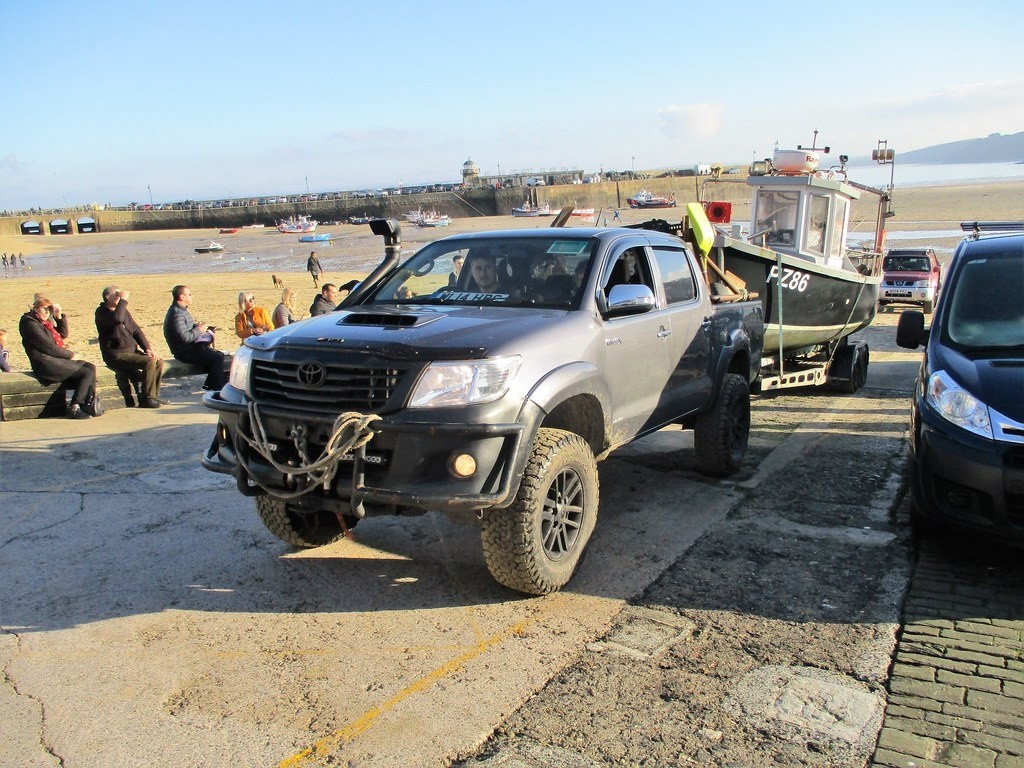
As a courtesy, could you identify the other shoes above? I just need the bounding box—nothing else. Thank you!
[202,385,209,390]
[155,397,171,405]
[66,407,93,419]
[140,399,162,408]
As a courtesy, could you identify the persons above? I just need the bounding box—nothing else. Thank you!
[163,285,226,390]
[572,260,608,305]
[604,216,608,227]
[307,252,323,288]
[234,292,274,344]
[11,254,16,267]
[468,249,510,294]
[2,253,8,267]
[613,211,621,222]
[19,292,97,418]
[271,287,305,329]
[94,286,168,408]
[0,329,13,372]
[448,255,464,286]
[400,286,414,299]
[19,253,26,266]
[310,283,337,317]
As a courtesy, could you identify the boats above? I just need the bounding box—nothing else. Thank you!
[512,198,595,217]
[681,128,895,360]
[349,214,390,225]
[219,225,265,234]
[626,191,676,209]
[298,233,331,242]
[277,214,318,233]
[195,241,225,254]
[401,206,452,227]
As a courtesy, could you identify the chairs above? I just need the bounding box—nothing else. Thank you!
[544,274,581,294]
[605,259,631,298]
[496,257,534,297]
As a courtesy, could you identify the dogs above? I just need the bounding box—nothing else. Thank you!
[339,280,361,295]
[272,275,284,288]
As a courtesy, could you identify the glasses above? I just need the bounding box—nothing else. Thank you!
[184,294,192,296]
[246,296,255,304]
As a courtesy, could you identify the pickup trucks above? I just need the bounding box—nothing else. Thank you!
[201,226,767,597]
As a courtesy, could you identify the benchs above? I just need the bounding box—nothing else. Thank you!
[904,261,927,269]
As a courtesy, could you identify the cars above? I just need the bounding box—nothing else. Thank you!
[895,222,1024,556]
[141,184,459,210]
[726,168,740,174]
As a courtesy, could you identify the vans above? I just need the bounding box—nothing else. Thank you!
[527,177,546,187]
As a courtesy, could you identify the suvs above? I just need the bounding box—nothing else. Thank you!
[878,248,942,314]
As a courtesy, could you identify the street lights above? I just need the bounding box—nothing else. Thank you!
[305,175,309,193]
[498,162,500,176]
[632,155,635,171]
[753,151,756,162]
[148,184,153,205]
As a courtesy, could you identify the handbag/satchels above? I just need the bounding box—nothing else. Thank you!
[194,329,216,352]
[80,393,103,417]
